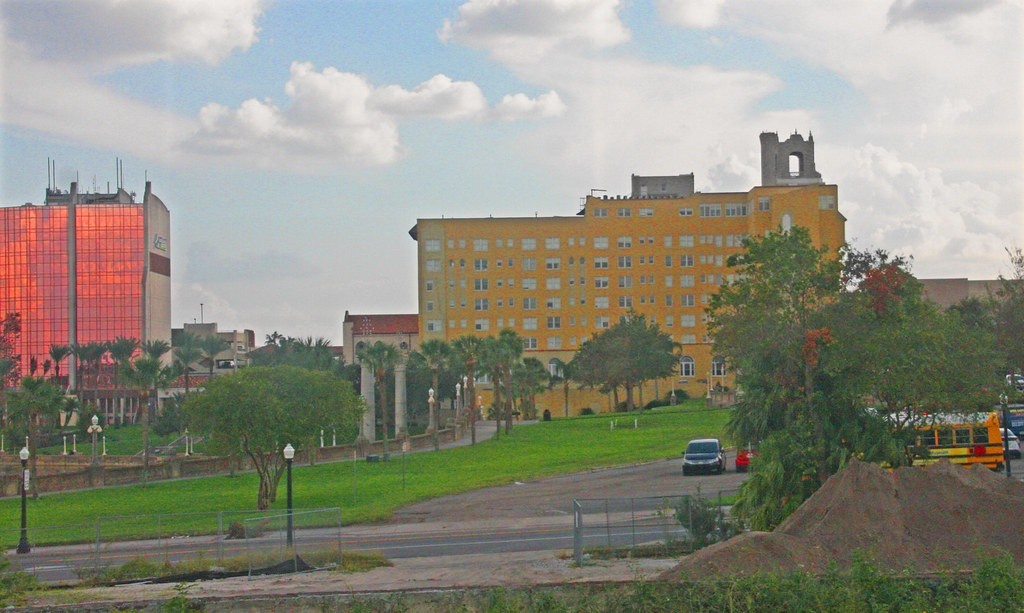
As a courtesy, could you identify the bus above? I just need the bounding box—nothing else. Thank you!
[800,411,1004,480]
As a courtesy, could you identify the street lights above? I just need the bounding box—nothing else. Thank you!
[283,443,295,550]
[669,371,676,406]
[16,447,31,554]
[999,390,1012,477]
[455,383,461,418]
[87,415,103,464]
[427,388,435,432]
[704,372,713,407]
[462,375,468,408]
[478,395,482,421]
[473,377,476,407]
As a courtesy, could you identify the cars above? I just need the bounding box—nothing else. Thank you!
[1006,374,1024,389]
[696,378,706,383]
[678,380,688,383]
[1000,428,1021,459]
[682,439,726,475]
[735,441,762,474]
